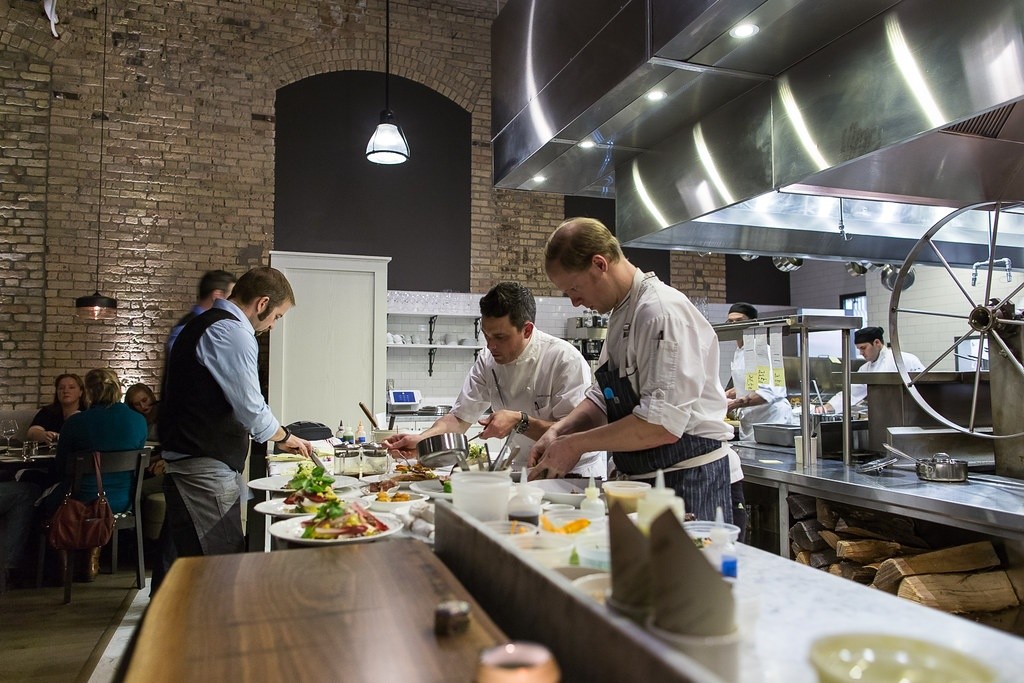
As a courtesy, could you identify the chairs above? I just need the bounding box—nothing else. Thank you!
[35,447,152,603]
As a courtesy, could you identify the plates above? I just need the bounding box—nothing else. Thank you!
[461,338,477,346]
[245,473,452,543]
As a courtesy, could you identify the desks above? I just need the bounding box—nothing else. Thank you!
[0,440,160,480]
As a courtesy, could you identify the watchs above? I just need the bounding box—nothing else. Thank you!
[275,426,292,443]
[516,411,530,434]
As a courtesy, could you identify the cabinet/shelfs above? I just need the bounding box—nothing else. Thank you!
[387,311,483,377]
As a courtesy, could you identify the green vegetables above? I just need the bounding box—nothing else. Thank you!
[288,466,345,538]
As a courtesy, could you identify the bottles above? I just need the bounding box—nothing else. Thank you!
[576,309,610,354]
[446,333,458,345]
[635,469,685,534]
[470,640,563,683]
[336,420,367,444]
[508,466,540,526]
[580,476,605,513]
[704,506,741,586]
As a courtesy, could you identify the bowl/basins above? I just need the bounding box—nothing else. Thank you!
[479,504,613,610]
[526,478,605,509]
[808,630,996,683]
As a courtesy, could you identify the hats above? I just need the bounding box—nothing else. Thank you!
[855,327,884,344]
[728,303,758,319]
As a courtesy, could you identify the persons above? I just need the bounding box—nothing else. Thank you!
[382,282,607,482]
[813,327,929,416]
[723,302,794,444]
[527,217,748,544]
[13,266,313,602]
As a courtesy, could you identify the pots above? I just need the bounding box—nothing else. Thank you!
[916,452,968,483]
[416,432,481,467]
[799,412,853,437]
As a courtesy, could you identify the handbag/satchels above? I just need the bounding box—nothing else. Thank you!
[45,451,116,550]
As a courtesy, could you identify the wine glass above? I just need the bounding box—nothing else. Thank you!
[0,419,19,457]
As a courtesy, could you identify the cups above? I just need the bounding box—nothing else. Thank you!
[602,480,651,513]
[22,440,39,462]
[387,332,420,344]
[683,520,741,550]
[49,433,59,449]
[450,471,511,523]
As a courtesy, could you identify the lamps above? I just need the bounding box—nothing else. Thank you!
[75,0,116,320]
[366,0,409,165]
